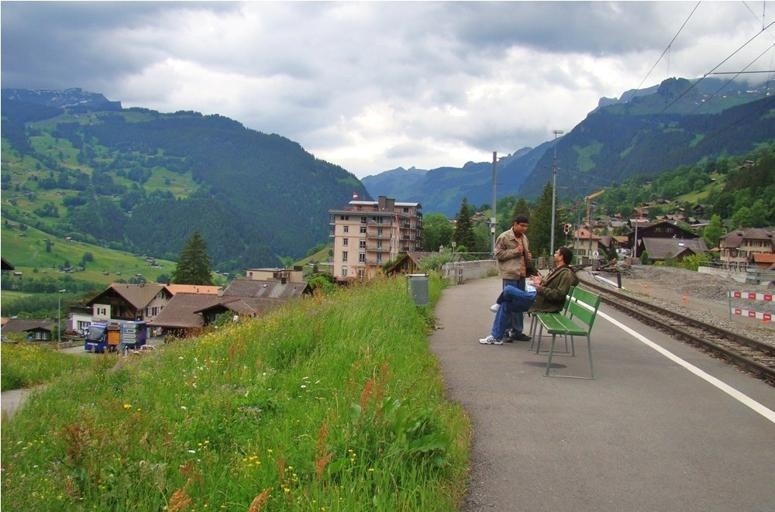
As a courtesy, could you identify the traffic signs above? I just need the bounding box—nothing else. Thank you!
[122,323,136,343]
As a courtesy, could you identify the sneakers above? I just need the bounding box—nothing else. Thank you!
[490,303,501,312]
[504,332,532,343]
[479,335,503,345]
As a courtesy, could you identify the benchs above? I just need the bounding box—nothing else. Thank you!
[527,285,600,379]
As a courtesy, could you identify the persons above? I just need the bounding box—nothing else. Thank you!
[479,247,577,345]
[525,249,541,277]
[493,215,534,342]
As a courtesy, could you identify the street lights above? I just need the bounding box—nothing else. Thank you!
[551,130,564,255]
[58,289,65,342]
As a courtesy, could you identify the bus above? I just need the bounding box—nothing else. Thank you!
[83,319,146,352]
